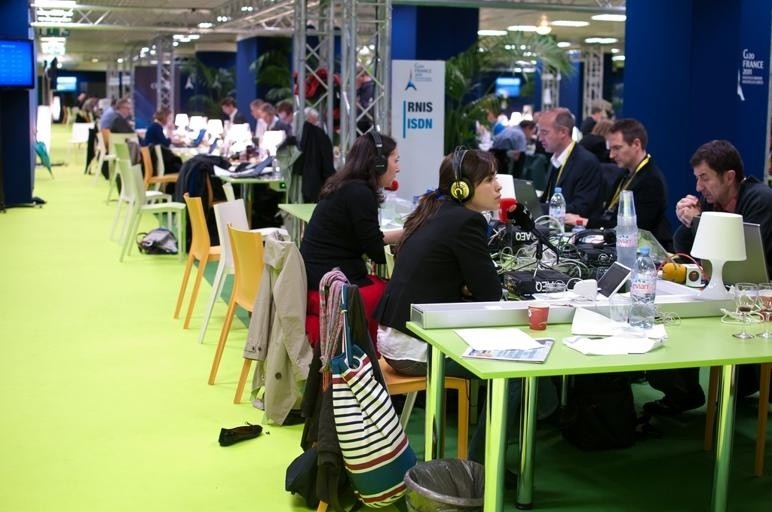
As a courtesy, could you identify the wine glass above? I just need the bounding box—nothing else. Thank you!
[732,283,772,340]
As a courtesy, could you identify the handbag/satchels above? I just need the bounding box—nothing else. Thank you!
[286,344,419,508]
[135,227,179,254]
[558,375,637,451]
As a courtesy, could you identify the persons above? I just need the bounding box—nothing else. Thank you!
[372,150,559,488]
[564,119,675,254]
[75,91,144,193]
[297,132,407,416]
[537,107,609,220]
[473,107,615,179]
[142,104,184,203]
[219,97,325,171]
[643,140,772,419]
[292,66,374,137]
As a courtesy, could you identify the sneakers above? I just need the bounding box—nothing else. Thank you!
[644,383,704,414]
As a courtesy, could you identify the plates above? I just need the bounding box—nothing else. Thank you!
[533,292,578,305]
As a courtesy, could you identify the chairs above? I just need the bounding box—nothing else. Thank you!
[172,192,220,329]
[264,229,320,416]
[704,362,772,476]
[199,199,250,344]
[210,223,268,406]
[315,266,467,512]
[65,104,291,264]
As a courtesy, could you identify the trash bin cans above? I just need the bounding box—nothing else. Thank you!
[402,456,485,512]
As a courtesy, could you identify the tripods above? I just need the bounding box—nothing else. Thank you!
[511,242,564,281]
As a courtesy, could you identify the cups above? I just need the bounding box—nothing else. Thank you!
[616,190,637,225]
[608,295,630,323]
[528,305,549,330]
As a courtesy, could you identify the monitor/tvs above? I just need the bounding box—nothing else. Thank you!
[0,34,36,92]
[54,75,78,92]
[492,75,523,99]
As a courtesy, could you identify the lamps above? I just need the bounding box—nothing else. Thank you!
[536,15,552,34]
[690,212,745,299]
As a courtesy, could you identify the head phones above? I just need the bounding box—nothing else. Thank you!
[447,145,475,204]
[367,130,388,176]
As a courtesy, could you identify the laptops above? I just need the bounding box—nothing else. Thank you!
[700,221,770,286]
[495,174,516,200]
[514,178,546,225]
[173,127,207,148]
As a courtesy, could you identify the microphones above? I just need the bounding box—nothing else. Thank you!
[506,203,562,256]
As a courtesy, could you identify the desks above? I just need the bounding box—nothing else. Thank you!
[277,194,418,279]
[406,316,772,512]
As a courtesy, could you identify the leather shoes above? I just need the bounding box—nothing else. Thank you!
[218,425,262,446]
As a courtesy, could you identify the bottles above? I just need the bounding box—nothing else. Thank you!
[549,188,567,235]
[630,248,657,326]
[572,220,587,243]
[616,217,638,271]
[272,157,281,179]
